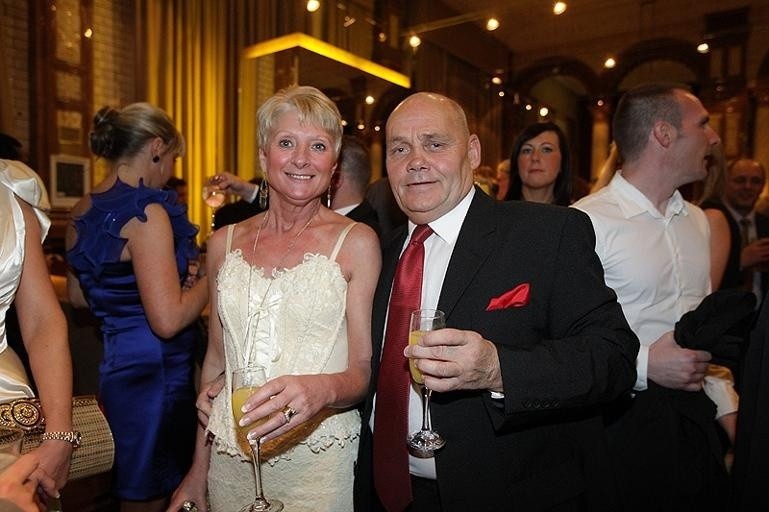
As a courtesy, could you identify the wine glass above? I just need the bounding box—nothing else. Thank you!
[404,308,446,458]
[201,176,226,237]
[230,366,284,512]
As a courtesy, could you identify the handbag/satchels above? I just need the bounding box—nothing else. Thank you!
[0,396,114,480]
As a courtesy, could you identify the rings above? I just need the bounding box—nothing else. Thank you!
[281,405,296,423]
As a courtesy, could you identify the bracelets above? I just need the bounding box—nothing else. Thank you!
[41,428,82,450]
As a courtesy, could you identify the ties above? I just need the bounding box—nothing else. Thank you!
[373,225,434,511]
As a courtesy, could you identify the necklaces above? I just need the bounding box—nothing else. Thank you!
[246,208,317,321]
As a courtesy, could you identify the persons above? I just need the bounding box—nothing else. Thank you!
[566,81,739,511]
[62,100,209,511]
[678,147,769,310]
[353,90,641,512]
[1,154,75,512]
[165,81,385,512]
[164,174,188,206]
[0,133,25,163]
[211,135,382,236]
[474,121,623,210]
